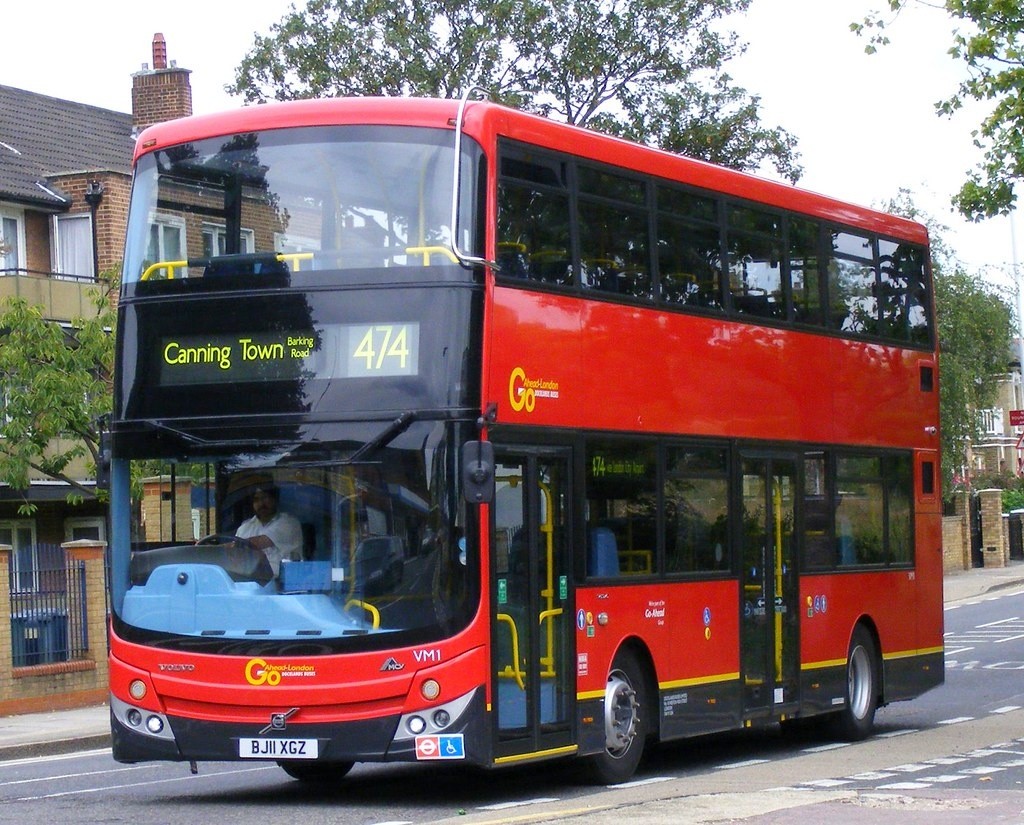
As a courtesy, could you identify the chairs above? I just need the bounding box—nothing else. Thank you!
[731,287,770,317]
[768,292,801,322]
[300,521,317,560]
[496,241,530,279]
[701,279,733,309]
[528,251,573,286]
[662,273,698,306]
[798,299,823,326]
[582,259,619,292]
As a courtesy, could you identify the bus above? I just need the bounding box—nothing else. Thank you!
[97,97,949,784]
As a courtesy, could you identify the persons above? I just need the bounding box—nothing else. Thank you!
[638,222,705,288]
[420,516,466,632]
[704,522,747,570]
[536,217,600,285]
[846,542,881,563]
[195,484,304,593]
[633,533,668,572]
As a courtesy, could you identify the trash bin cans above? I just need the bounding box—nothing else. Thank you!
[11,607,68,665]
